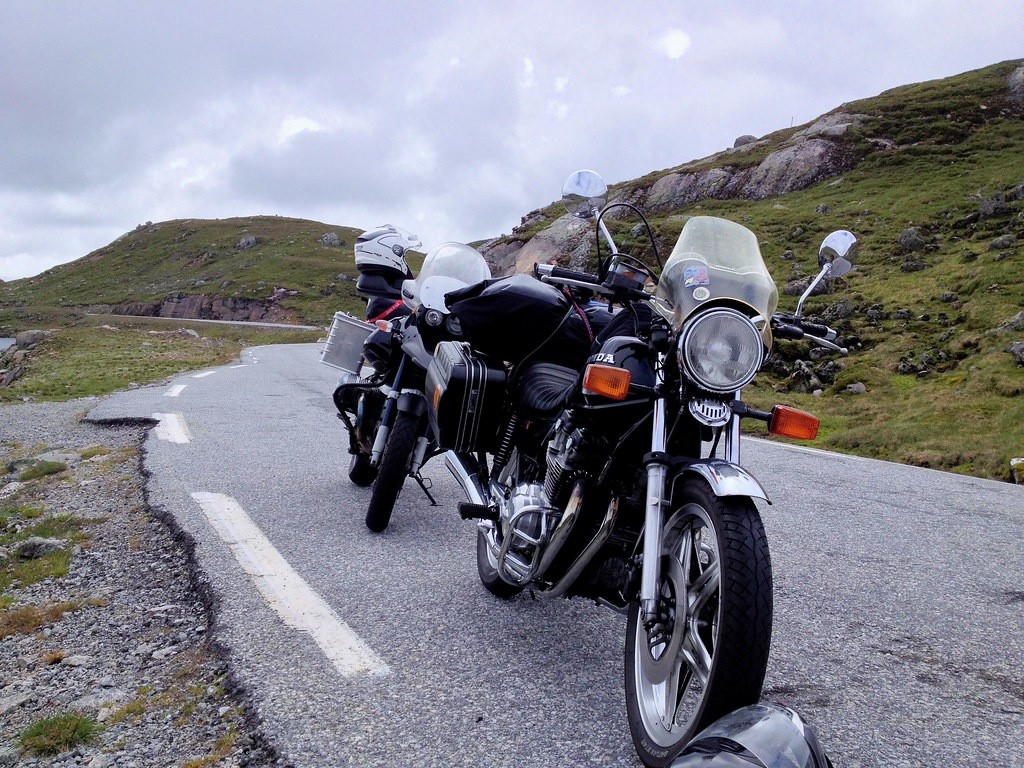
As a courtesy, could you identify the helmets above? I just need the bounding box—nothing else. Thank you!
[664,703,834,768]
[354,223,422,275]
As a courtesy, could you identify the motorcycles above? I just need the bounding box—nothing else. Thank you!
[321,243,493,533]
[423,171,859,768]
